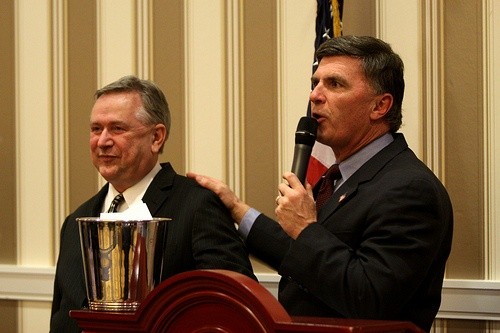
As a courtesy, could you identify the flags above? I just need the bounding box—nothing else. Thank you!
[305,1,344,187]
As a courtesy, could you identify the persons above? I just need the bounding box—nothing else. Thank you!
[187,35,454,333]
[43,76,257,333]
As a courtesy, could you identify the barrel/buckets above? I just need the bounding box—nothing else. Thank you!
[77,217,171,312]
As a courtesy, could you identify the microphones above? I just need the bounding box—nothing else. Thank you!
[288,117,318,189]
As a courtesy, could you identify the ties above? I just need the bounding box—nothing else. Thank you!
[316,164,342,216]
[108,194,125,213]
